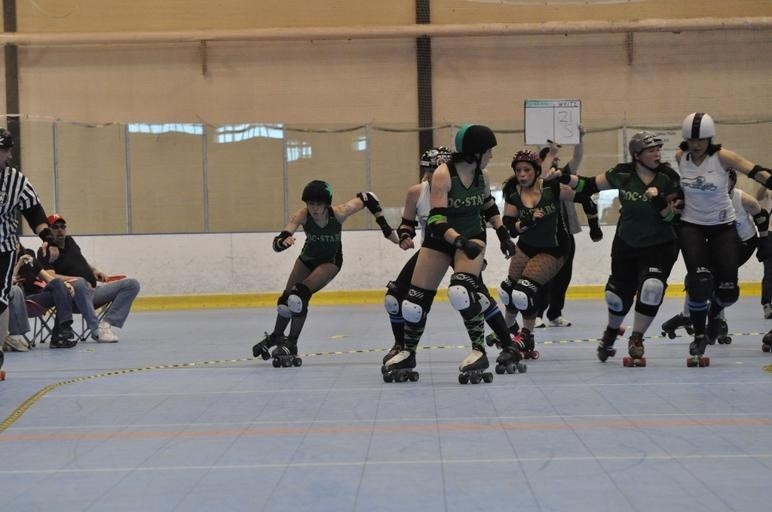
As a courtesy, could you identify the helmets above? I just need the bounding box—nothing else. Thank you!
[419,123,497,168]
[728,168,737,187]
[628,132,664,157]
[0,128,14,149]
[302,180,332,205]
[511,150,542,168]
[683,112,716,140]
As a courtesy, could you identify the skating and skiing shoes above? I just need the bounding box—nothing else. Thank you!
[459,347,492,385]
[486,322,540,373]
[623,330,647,368]
[661,303,731,344]
[253,333,302,367]
[380,344,419,382]
[685,333,710,368]
[597,323,625,365]
[762,331,772,353]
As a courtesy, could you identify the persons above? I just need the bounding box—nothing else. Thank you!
[1,126,56,381]
[35,213,141,344]
[255,180,399,357]
[11,235,79,349]
[3,283,32,353]
[382,111,770,383]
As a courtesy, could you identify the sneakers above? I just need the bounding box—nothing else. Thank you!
[5,333,30,352]
[538,315,571,327]
[763,303,771,319]
[50,328,77,348]
[92,322,119,344]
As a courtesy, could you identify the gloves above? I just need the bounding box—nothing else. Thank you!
[757,247,768,262]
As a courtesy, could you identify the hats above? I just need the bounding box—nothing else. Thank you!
[48,214,66,225]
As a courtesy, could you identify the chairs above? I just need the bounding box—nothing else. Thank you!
[23,275,128,350]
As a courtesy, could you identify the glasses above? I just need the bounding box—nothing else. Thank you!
[50,225,67,229]
